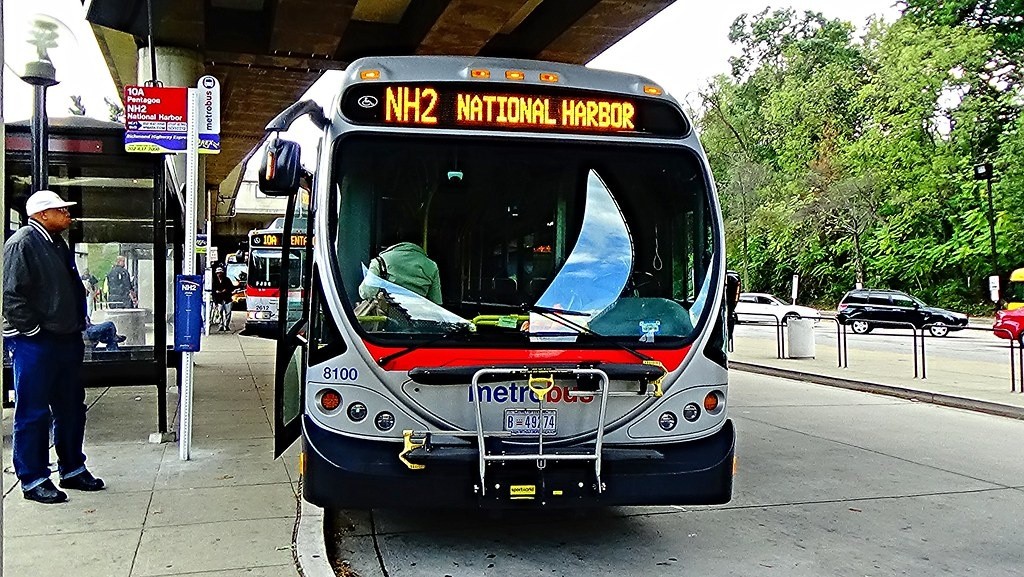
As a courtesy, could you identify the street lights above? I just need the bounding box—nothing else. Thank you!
[974,163,1001,312]
[18,19,61,458]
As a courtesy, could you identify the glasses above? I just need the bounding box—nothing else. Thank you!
[59,206,69,212]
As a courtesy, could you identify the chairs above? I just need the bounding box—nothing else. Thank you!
[464,250,553,305]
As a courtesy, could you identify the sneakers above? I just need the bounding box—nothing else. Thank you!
[24,479,67,504]
[59,469,105,490]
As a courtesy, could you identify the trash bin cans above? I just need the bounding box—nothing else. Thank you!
[787,318,816,359]
[106,308,146,345]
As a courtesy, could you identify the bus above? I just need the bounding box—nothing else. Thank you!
[225,251,246,312]
[999,268,1024,310]
[235,218,309,332]
[259,54,742,508]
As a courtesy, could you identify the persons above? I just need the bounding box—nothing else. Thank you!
[3,190,105,504]
[358,228,444,306]
[79,267,99,298]
[212,267,235,331]
[107,256,136,309]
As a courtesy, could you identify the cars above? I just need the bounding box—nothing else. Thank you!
[993,307,1023,347]
[733,293,822,327]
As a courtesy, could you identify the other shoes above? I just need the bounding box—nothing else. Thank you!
[116,334,127,343]
[225,327,232,332]
[218,327,224,332]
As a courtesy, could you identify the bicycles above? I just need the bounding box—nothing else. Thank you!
[210,300,219,325]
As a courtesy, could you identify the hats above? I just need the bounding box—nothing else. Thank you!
[25,190,78,217]
[215,266,224,273]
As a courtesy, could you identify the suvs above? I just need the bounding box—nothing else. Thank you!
[836,287,969,337]
